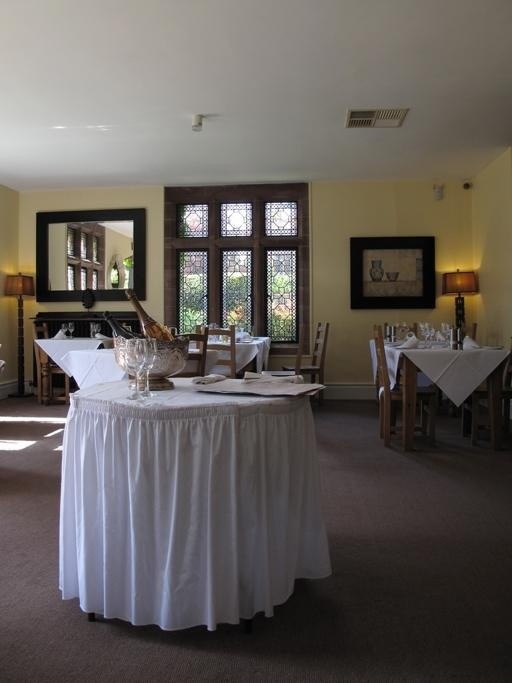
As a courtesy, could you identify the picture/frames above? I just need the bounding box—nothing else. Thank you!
[351,236,436,309]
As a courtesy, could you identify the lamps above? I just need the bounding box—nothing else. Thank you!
[442,268,480,328]
[4,272,35,398]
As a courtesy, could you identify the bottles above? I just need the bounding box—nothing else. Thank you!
[102,311,137,337]
[124,288,173,339]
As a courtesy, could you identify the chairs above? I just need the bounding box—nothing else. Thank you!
[369,322,512,452]
[36,321,329,410]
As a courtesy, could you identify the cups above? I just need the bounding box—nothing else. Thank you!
[90,322,101,337]
[386,272,399,282]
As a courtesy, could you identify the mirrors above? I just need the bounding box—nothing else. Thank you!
[36,208,146,302]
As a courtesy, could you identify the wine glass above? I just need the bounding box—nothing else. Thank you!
[419,321,450,350]
[239,323,245,332]
[62,322,75,339]
[125,338,157,401]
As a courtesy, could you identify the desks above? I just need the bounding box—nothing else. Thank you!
[58,377,332,633]
